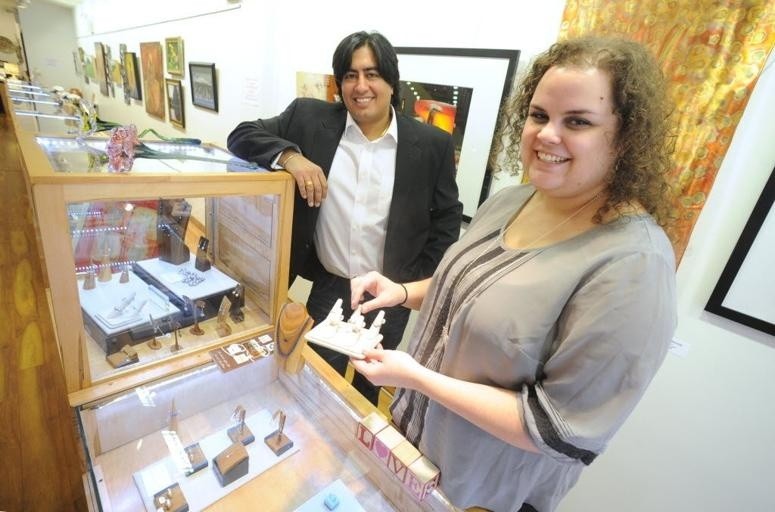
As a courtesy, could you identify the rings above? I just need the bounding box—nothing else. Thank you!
[306,182,312,186]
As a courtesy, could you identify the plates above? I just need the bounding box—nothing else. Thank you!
[303,318,384,361]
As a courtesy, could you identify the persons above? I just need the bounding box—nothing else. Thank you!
[351,34,676,511]
[226,33,463,406]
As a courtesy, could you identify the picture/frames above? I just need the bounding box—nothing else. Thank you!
[188,61,219,112]
[165,78,185,128]
[125,52,142,100]
[139,41,165,119]
[93,42,109,97]
[165,36,184,76]
[78,48,89,85]
[702,172,775,340]
[389,45,522,225]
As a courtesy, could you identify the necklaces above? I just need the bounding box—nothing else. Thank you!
[275,303,310,358]
[428,194,607,353]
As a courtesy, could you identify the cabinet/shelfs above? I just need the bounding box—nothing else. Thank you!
[3,77,297,408]
[75,341,461,512]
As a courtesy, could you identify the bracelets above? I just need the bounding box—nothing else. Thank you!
[281,153,303,170]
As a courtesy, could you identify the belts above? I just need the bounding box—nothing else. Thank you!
[321,271,350,288]
